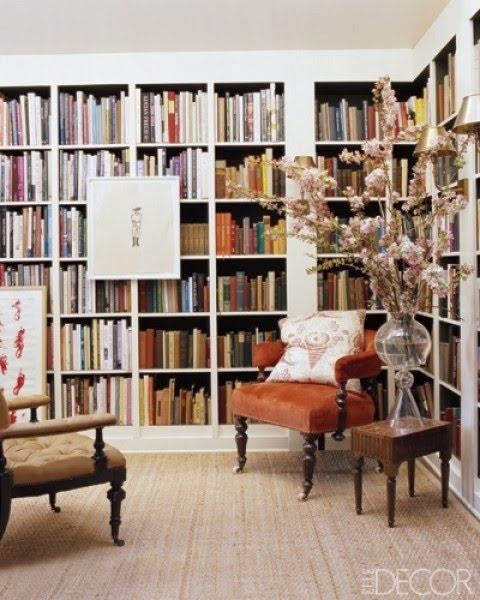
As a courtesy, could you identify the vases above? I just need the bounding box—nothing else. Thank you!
[374,313,432,434]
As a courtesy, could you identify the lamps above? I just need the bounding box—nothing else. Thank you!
[412,94,480,203]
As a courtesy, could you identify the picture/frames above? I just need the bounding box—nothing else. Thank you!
[0,285,46,424]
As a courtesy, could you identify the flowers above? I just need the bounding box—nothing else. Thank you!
[232,76,476,364]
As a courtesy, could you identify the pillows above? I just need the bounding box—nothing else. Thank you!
[265,308,367,395]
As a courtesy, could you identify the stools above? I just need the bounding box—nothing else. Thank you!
[350,415,453,527]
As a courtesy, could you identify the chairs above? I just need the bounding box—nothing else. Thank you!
[231,331,379,503]
[0,392,126,547]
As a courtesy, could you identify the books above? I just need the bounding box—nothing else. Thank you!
[1,38,480,483]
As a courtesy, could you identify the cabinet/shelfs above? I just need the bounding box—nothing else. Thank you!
[316,9,479,519]
[0,82,284,458]
